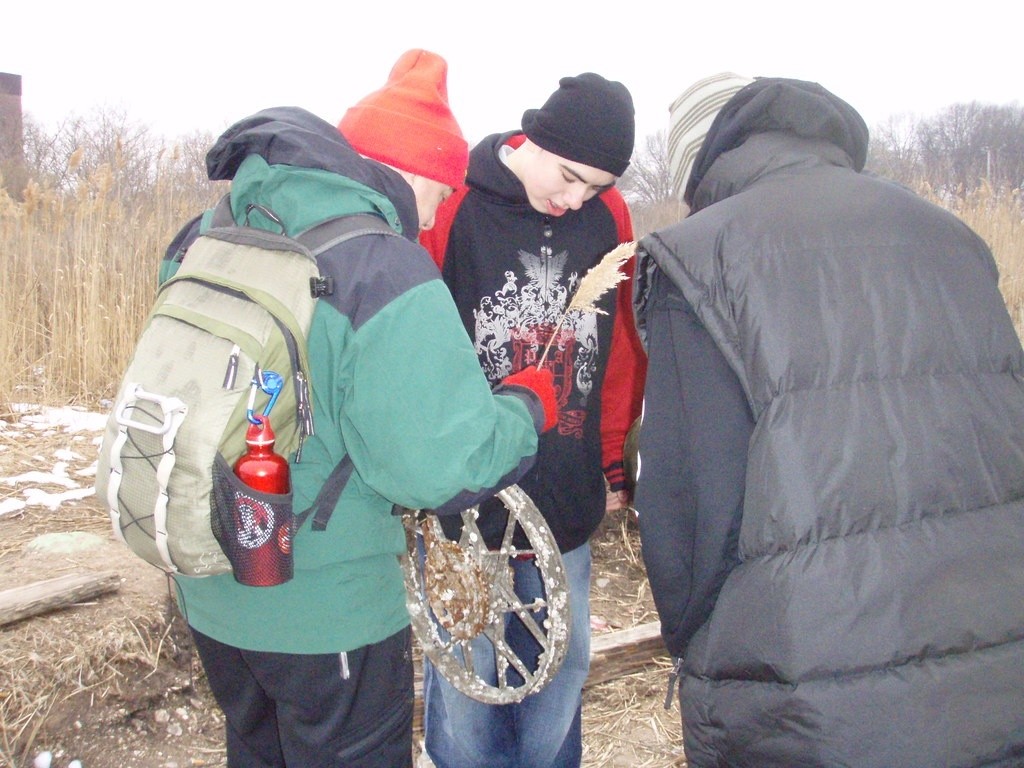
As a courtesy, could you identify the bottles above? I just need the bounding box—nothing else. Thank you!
[232,415,294,587]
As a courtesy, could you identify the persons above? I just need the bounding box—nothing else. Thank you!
[418,72,648,768]
[633,72,1024,768]
[159,50,559,768]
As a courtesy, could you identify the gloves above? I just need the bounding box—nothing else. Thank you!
[502,365,559,434]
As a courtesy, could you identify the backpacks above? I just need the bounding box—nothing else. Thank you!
[95,192,412,587]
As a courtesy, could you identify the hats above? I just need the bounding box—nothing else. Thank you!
[667,73,758,203]
[336,49,470,191]
[521,73,636,177]
